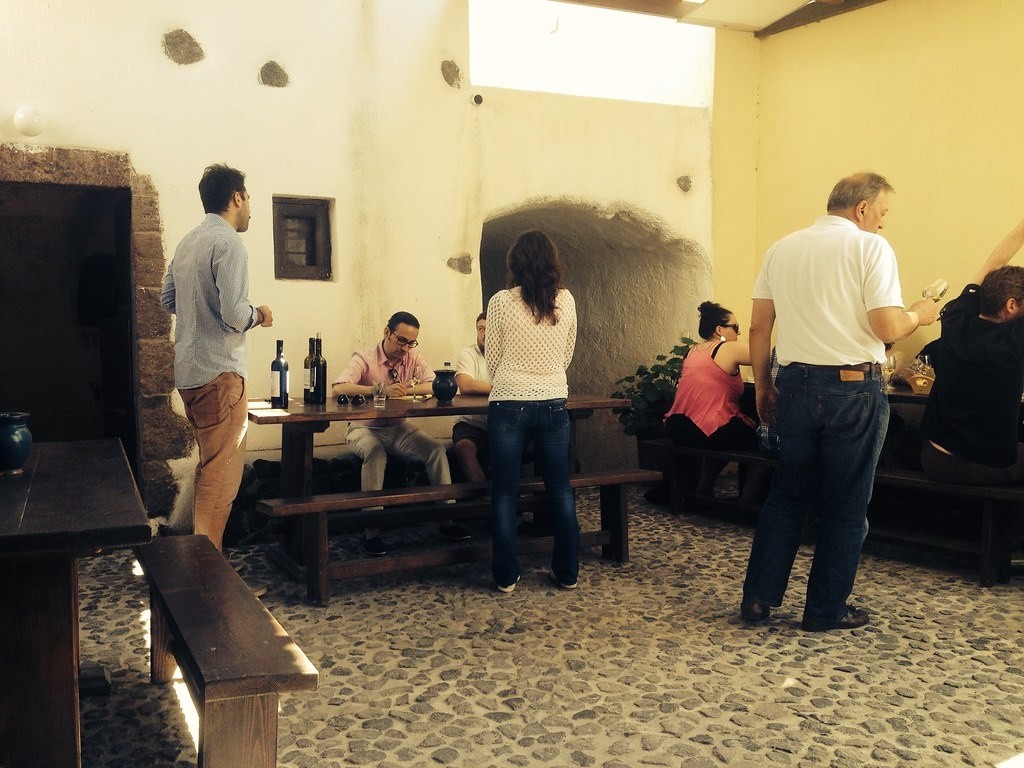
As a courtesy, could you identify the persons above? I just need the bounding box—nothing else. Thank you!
[737,172,937,630]
[450,311,496,486]
[483,230,583,591]
[910,260,1024,564]
[658,299,758,505]
[331,311,474,555]
[158,165,274,599]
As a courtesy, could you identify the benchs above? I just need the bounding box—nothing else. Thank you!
[132,534,320,766]
[236,455,664,603]
[642,435,1024,583]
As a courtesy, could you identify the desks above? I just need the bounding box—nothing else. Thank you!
[0,437,152,767]
[739,376,1024,470]
[244,394,630,584]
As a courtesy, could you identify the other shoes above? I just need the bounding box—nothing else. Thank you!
[245,582,267,598]
[739,597,772,622]
[360,532,388,556]
[438,523,473,539]
[498,573,521,593]
[547,568,579,589]
[800,607,872,632]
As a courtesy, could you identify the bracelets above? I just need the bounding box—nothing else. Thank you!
[256,307,266,322]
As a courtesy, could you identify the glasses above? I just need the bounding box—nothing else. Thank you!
[723,322,740,334]
[390,329,419,349]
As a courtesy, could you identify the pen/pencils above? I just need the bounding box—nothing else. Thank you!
[396,375,408,397]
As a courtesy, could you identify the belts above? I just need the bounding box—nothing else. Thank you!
[792,361,883,374]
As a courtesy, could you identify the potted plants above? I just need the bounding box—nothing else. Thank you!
[610,335,697,504]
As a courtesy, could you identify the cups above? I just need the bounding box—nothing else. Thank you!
[373,381,387,408]
[923,278,949,303]
[917,355,931,376]
[747,365,755,383]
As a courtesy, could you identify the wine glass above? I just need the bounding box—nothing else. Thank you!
[408,365,422,403]
[880,356,896,390]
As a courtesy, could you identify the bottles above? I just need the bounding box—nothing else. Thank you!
[432,370,458,404]
[443,362,450,369]
[309,332,327,403]
[304,338,315,402]
[270,340,289,409]
